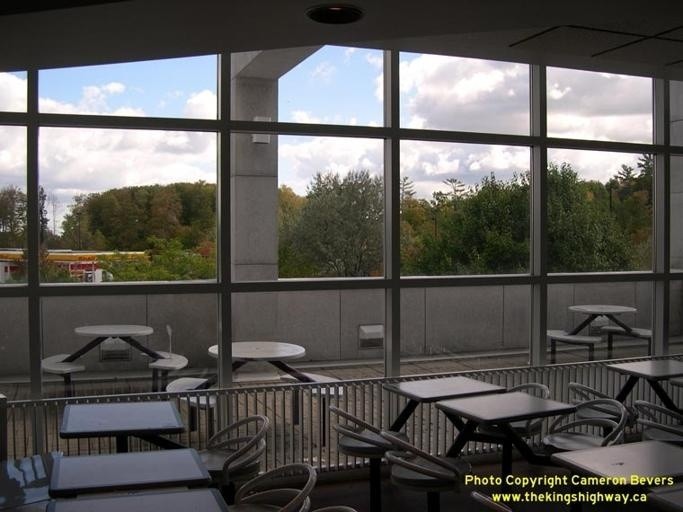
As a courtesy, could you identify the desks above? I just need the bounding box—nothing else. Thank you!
[568,303,637,334]
[605,361,682,410]
[208,341,315,384]
[103,324,160,358]
[553,440,682,512]
[102,402,230,512]
[382,376,579,461]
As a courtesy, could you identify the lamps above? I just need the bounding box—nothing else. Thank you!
[305,3,368,28]
